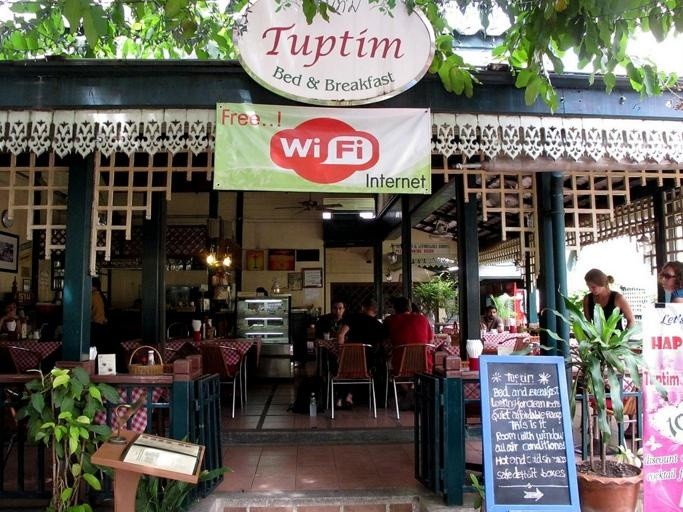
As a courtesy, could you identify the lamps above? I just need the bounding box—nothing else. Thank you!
[204,215,220,268]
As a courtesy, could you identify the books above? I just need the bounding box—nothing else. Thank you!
[120,432,202,476]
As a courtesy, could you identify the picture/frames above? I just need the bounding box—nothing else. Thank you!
[0,231,23,275]
[287,273,304,292]
[303,267,326,289]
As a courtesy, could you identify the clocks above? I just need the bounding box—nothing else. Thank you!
[1,207,15,229]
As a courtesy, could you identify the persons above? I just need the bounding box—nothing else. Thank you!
[481,305,504,333]
[90,277,106,347]
[336,297,384,408]
[0,296,21,331]
[383,296,434,380]
[658,260,683,303]
[583,269,635,337]
[314,300,346,343]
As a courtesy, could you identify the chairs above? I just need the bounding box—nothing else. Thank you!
[0,343,47,479]
[162,347,187,364]
[330,343,379,420]
[199,346,244,419]
[384,344,435,420]
[17,406,18,408]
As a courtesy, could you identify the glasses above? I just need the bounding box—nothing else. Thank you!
[659,272,678,279]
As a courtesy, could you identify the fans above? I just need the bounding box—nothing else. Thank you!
[273,192,344,216]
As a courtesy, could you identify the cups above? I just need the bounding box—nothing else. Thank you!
[323,332,330,341]
[491,328,497,333]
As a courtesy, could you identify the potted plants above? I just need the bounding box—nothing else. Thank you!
[507,279,645,512]
